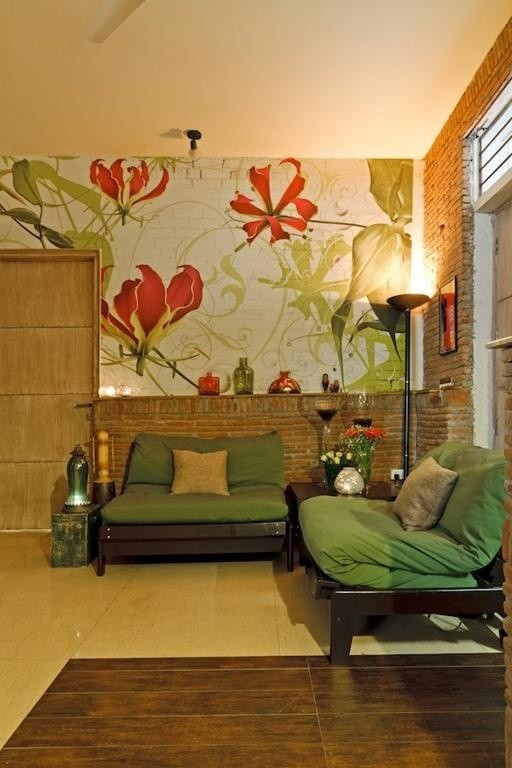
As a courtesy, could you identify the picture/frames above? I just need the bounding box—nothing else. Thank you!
[439,276,457,355]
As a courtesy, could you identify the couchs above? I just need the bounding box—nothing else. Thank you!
[298,441,505,664]
[99,431,292,575]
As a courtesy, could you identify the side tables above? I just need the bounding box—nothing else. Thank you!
[50,505,99,567]
[286,481,401,572]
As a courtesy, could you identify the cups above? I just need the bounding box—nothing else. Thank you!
[353,405,372,429]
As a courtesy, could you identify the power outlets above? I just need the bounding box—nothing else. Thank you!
[391,469,404,481]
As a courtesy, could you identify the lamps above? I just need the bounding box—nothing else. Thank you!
[315,407,337,486]
[387,294,431,478]
[186,130,202,160]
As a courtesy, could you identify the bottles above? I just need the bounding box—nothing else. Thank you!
[233,356,253,394]
[198,371,220,395]
[267,370,302,393]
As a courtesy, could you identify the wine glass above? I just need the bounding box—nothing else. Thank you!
[314,394,338,488]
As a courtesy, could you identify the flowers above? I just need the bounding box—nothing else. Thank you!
[319,424,388,494]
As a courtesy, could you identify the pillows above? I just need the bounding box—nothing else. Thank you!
[392,456,459,532]
[169,449,230,497]
